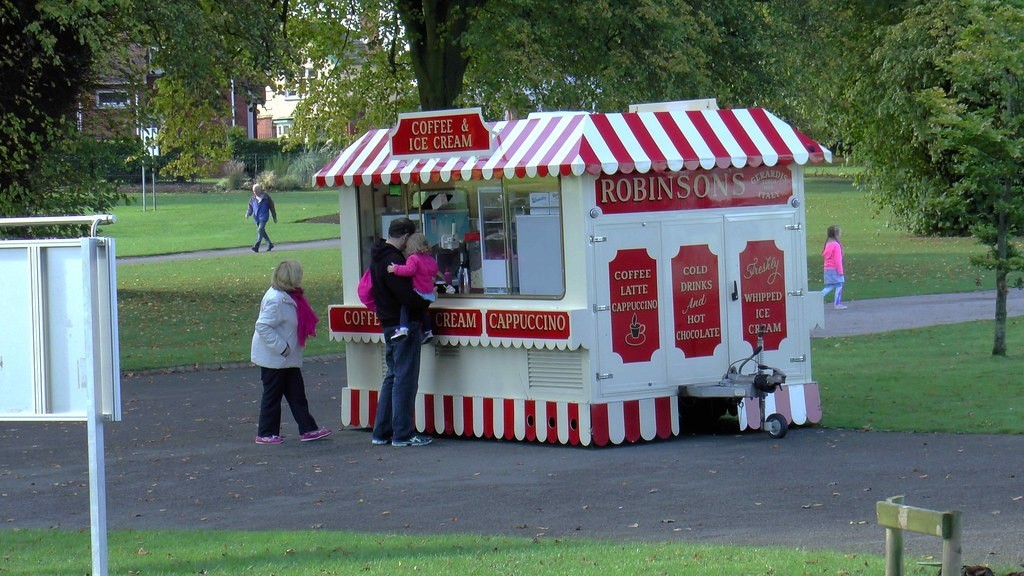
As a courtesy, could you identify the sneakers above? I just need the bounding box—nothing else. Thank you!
[391,328,407,342]
[420,330,434,344]
[392,432,433,447]
[371,434,391,445]
[256,434,283,444]
[300,427,332,442]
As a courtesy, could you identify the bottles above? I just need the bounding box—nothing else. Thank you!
[457,266,463,294]
[443,268,452,286]
[461,267,471,294]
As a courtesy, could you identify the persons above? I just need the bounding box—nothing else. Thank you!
[428,237,471,281]
[368,217,448,448]
[244,183,278,253]
[819,225,849,310]
[250,259,333,445]
[386,232,439,345]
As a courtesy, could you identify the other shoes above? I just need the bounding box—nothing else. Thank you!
[251,247,259,252]
[833,304,848,310]
[267,244,274,251]
